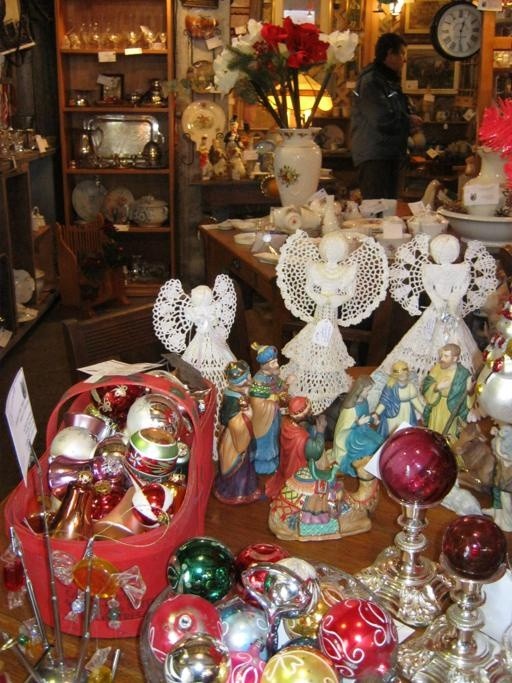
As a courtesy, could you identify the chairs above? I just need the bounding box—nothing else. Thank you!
[62,285,253,381]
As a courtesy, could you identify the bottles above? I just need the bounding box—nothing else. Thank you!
[321,194,338,238]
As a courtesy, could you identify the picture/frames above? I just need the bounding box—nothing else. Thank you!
[401,0,453,43]
[400,42,460,95]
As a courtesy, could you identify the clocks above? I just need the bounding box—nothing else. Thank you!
[429,1,483,62]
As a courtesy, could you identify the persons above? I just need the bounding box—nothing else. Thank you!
[213,342,476,544]
[348,33,424,200]
[196,119,244,181]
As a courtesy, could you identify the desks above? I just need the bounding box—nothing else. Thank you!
[189,173,336,222]
[196,215,465,364]
[1,364,512,683]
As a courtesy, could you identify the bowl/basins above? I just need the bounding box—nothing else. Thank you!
[435,207,512,248]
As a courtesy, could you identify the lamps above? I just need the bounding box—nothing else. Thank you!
[268,72,334,128]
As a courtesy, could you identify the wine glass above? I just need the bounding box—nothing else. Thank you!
[79,22,166,50]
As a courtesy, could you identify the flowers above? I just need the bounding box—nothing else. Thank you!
[205,16,358,129]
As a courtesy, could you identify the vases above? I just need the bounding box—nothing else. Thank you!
[461,146,508,216]
[270,127,324,208]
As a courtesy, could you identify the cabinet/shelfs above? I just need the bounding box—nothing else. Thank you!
[313,118,474,204]
[1,139,59,359]
[55,1,177,296]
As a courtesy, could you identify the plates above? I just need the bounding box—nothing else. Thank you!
[102,188,134,223]
[182,100,227,146]
[187,62,215,93]
[72,180,105,223]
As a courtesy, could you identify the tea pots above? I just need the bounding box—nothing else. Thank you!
[121,194,169,228]
[69,126,104,167]
